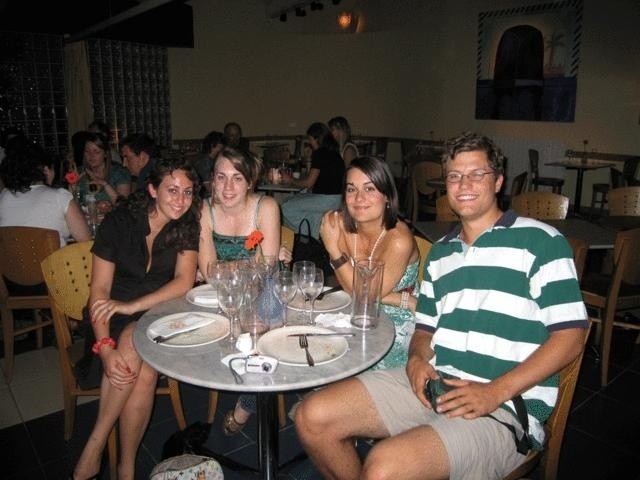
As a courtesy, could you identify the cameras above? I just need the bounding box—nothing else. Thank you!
[244,355,278,375]
[424,370,462,415]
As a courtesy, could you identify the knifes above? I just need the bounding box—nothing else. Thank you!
[289,333,356,338]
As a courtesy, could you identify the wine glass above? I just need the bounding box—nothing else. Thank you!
[206,256,325,345]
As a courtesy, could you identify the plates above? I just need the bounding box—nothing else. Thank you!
[186,282,225,307]
[258,326,348,365]
[147,312,228,347]
[288,287,351,314]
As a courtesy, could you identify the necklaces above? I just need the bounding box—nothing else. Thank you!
[354,225,385,268]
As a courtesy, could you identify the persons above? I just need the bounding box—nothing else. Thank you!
[294,132,589,480]
[69,152,201,480]
[317,155,421,375]
[195,147,292,438]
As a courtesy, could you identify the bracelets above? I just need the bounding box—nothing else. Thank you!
[92,337,116,354]
[400,291,409,310]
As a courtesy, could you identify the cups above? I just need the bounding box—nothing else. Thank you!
[82,194,105,222]
[267,158,300,185]
[352,256,386,330]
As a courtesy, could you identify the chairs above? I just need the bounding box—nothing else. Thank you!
[3,226,63,384]
[415,235,434,289]
[41,240,186,476]
[507,316,592,478]
[382,140,460,232]
[509,148,640,387]
[206,226,295,426]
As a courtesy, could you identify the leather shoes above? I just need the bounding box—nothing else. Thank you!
[222,397,252,437]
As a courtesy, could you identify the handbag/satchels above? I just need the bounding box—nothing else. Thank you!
[290,218,322,273]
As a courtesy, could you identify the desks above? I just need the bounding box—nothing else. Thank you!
[130,283,397,478]
[257,184,304,205]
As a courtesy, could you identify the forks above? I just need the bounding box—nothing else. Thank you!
[299,334,313,367]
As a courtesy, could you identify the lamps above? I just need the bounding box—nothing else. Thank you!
[278,1,340,24]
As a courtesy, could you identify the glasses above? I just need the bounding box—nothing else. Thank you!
[445,171,497,183]
